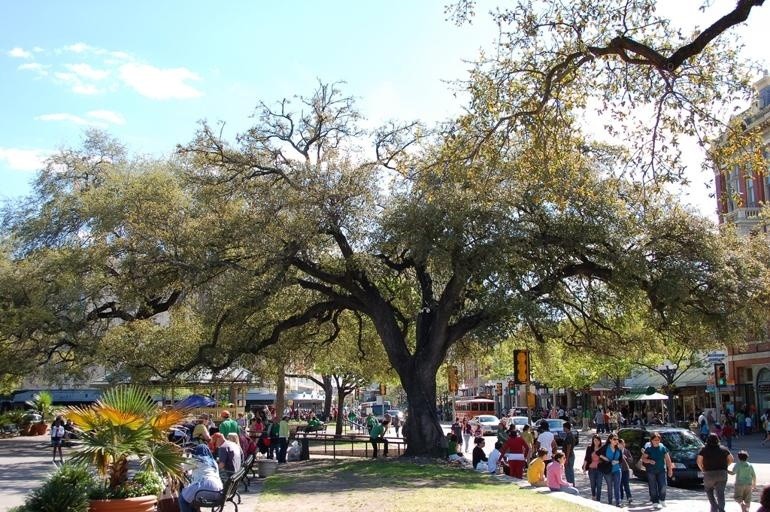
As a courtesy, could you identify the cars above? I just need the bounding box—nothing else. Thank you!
[384,408,404,427]
[467,414,501,437]
[165,420,196,443]
[19,414,43,422]
[533,417,580,447]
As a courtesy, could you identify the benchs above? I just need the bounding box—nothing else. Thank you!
[195,447,262,511]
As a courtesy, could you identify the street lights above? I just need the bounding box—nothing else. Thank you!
[659,356,677,424]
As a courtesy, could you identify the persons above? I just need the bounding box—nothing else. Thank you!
[698,408,769,445]
[327,401,405,437]
[545,450,582,496]
[618,438,635,505]
[563,422,575,487]
[49,400,326,512]
[697,433,734,511]
[592,405,630,433]
[597,432,624,508]
[583,434,603,501]
[641,432,673,510]
[370,420,391,460]
[727,450,757,512]
[472,414,558,487]
[451,418,483,453]
[541,407,578,425]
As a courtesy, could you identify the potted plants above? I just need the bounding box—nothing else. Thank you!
[8,408,34,436]
[61,381,192,512]
[30,389,53,435]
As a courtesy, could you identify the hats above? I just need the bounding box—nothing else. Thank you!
[221,410,230,417]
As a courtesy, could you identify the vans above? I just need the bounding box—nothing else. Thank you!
[614,426,707,488]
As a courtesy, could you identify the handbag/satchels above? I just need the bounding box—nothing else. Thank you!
[598,462,612,473]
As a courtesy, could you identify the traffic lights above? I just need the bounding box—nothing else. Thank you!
[355,389,359,399]
[713,362,728,388]
[721,394,730,403]
[509,381,515,395]
[497,383,502,396]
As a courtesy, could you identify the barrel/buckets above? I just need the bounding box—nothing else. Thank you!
[254,458,279,478]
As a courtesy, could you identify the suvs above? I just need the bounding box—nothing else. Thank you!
[497,414,539,442]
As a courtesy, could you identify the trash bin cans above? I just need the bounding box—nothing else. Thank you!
[289,437,309,461]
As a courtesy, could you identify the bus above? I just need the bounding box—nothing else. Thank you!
[238,391,327,420]
[358,400,392,425]
[455,394,496,430]
